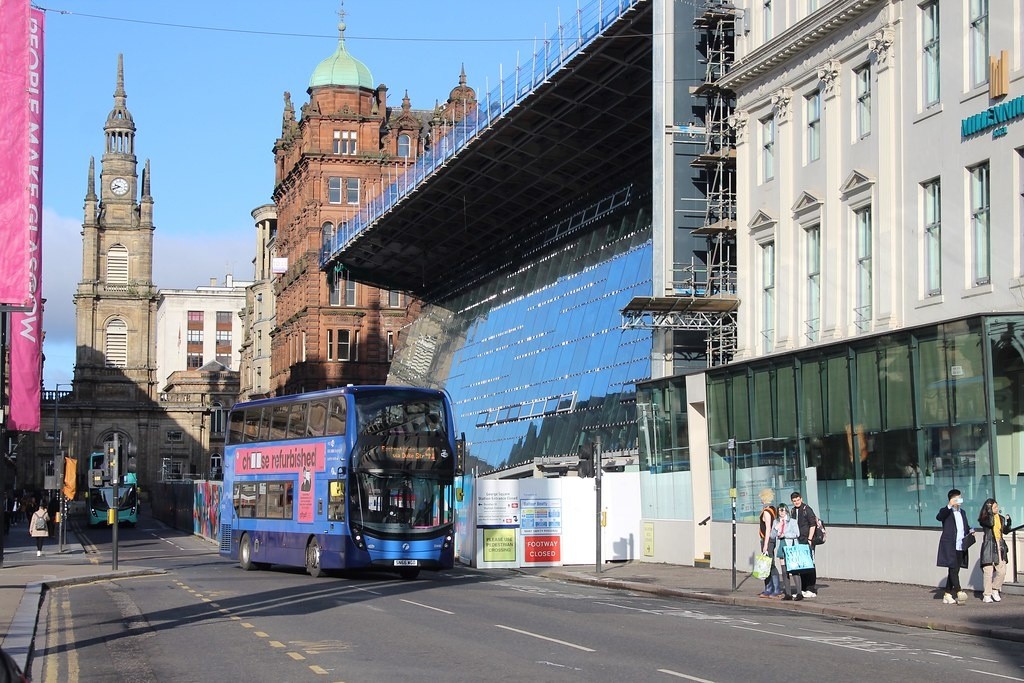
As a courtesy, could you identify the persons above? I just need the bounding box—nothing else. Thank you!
[301,467,310,491]
[936,489,975,606]
[757,489,818,601]
[978,498,1012,603]
[421,414,442,432]
[28,499,51,556]
[350,490,363,512]
[2,489,60,539]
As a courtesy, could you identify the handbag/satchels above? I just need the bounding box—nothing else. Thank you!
[752,551,772,581]
[782,539,814,571]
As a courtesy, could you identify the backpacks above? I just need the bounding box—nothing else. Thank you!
[792,505,827,544]
[34,512,46,530]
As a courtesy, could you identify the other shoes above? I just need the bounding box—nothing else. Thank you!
[759,593,769,597]
[792,591,805,597]
[794,594,803,601]
[768,594,783,598]
[37,550,41,557]
[780,594,793,600]
[803,591,817,598]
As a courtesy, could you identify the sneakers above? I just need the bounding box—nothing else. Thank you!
[991,590,1002,601]
[956,590,968,605]
[943,594,957,604]
[983,596,994,602]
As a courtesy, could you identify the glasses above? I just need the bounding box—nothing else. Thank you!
[777,508,786,512]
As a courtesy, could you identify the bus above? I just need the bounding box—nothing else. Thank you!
[83,451,143,529]
[216,381,466,579]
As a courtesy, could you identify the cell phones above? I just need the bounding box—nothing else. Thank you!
[957,498,963,503]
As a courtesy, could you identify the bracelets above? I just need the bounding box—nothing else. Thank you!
[808,539,812,541]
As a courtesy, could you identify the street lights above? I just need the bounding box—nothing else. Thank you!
[51,382,76,457]
